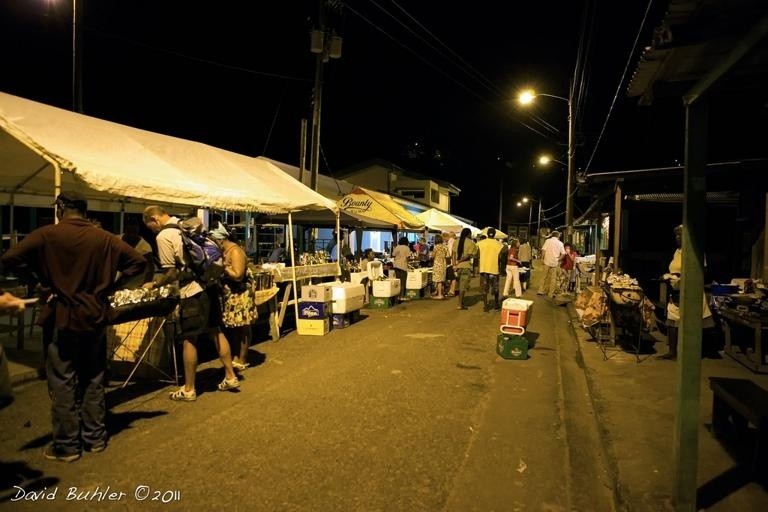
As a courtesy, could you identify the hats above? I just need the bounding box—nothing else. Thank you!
[49,191,87,210]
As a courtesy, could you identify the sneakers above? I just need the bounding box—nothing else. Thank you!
[537,293,543,296]
[44,440,106,461]
[663,352,676,359]
[444,291,456,297]
[549,294,556,298]
[169,384,196,402]
[456,303,468,310]
[217,377,240,390]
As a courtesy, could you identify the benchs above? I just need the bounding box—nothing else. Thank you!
[708,375,767,446]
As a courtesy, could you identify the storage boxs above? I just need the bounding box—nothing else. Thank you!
[497,333,528,360]
[293,262,431,338]
[498,296,534,328]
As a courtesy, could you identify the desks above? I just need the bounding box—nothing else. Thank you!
[110,295,181,389]
[276,261,340,329]
[599,279,643,362]
[253,286,282,343]
[572,257,598,290]
[718,307,767,373]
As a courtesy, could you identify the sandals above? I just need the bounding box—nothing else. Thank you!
[231,361,250,371]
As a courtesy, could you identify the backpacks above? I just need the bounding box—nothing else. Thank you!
[159,219,225,288]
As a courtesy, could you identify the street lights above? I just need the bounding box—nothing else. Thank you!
[536,153,576,221]
[516,201,533,245]
[522,196,542,249]
[517,89,575,249]
[496,154,504,232]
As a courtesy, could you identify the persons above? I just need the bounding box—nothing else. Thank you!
[269,243,287,263]
[657,225,715,360]
[1,190,147,462]
[392,228,577,312]
[141,205,240,402]
[360,248,375,287]
[0,293,26,409]
[115,237,155,291]
[207,228,258,370]
[324,229,344,282]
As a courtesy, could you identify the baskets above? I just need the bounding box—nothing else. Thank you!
[498,335,528,361]
